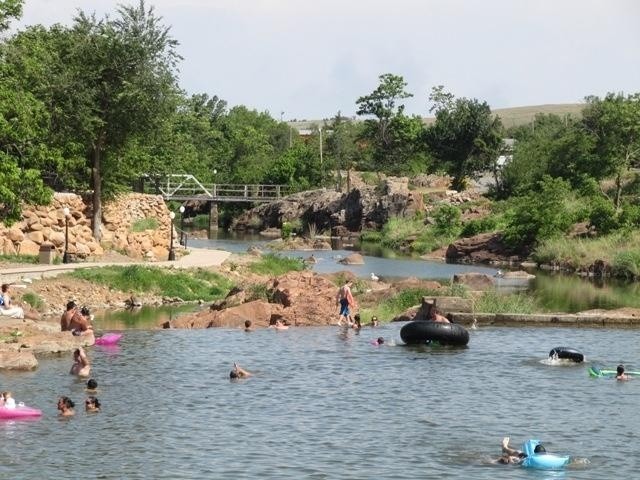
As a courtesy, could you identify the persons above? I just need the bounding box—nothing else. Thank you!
[83,380,101,393]
[85,396,101,413]
[370,335,385,345]
[350,313,364,328]
[0,390,16,408]
[494,268,502,278]
[70,306,94,337]
[68,346,91,377]
[367,316,379,326]
[57,395,76,416]
[243,319,258,333]
[58,300,77,331]
[269,318,289,330]
[334,278,354,326]
[488,436,549,467]
[426,308,453,325]
[307,253,316,262]
[615,365,632,382]
[0,282,25,321]
[467,318,477,330]
[228,360,254,378]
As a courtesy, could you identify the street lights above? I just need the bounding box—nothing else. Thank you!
[289,126,293,147]
[169,211,176,261]
[318,129,324,164]
[179,205,186,247]
[64,207,72,264]
[280,111,285,121]
[213,169,217,195]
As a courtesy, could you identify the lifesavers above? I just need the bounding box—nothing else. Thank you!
[95,333,121,345]
[549,346,584,363]
[587,365,639,379]
[521,439,571,471]
